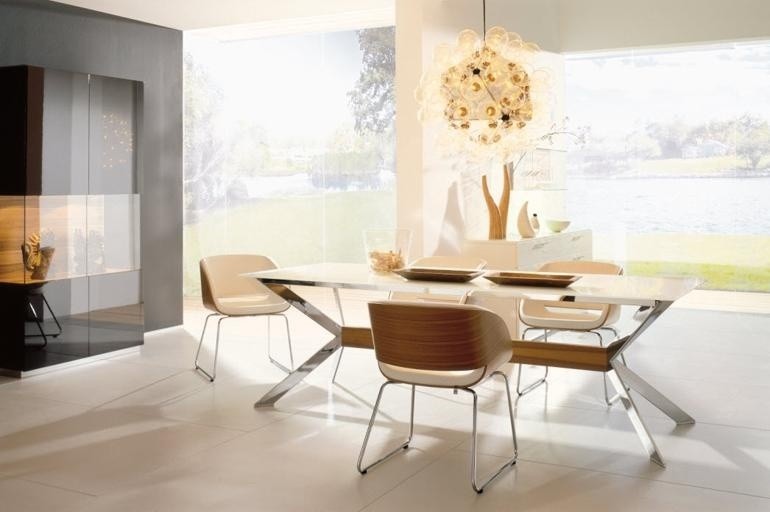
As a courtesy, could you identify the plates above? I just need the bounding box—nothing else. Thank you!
[392,267,582,287]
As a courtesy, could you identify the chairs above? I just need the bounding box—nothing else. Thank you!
[516,259,631,407]
[384,256,488,395]
[194,254,295,382]
[356,299,518,493]
[25,281,62,348]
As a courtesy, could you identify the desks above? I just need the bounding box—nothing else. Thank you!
[237,264,706,471]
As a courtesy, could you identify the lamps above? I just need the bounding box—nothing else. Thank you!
[413,0,555,167]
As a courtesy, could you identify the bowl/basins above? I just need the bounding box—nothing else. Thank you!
[546,220,571,234]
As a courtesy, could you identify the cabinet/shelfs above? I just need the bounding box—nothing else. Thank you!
[462,230,594,273]
